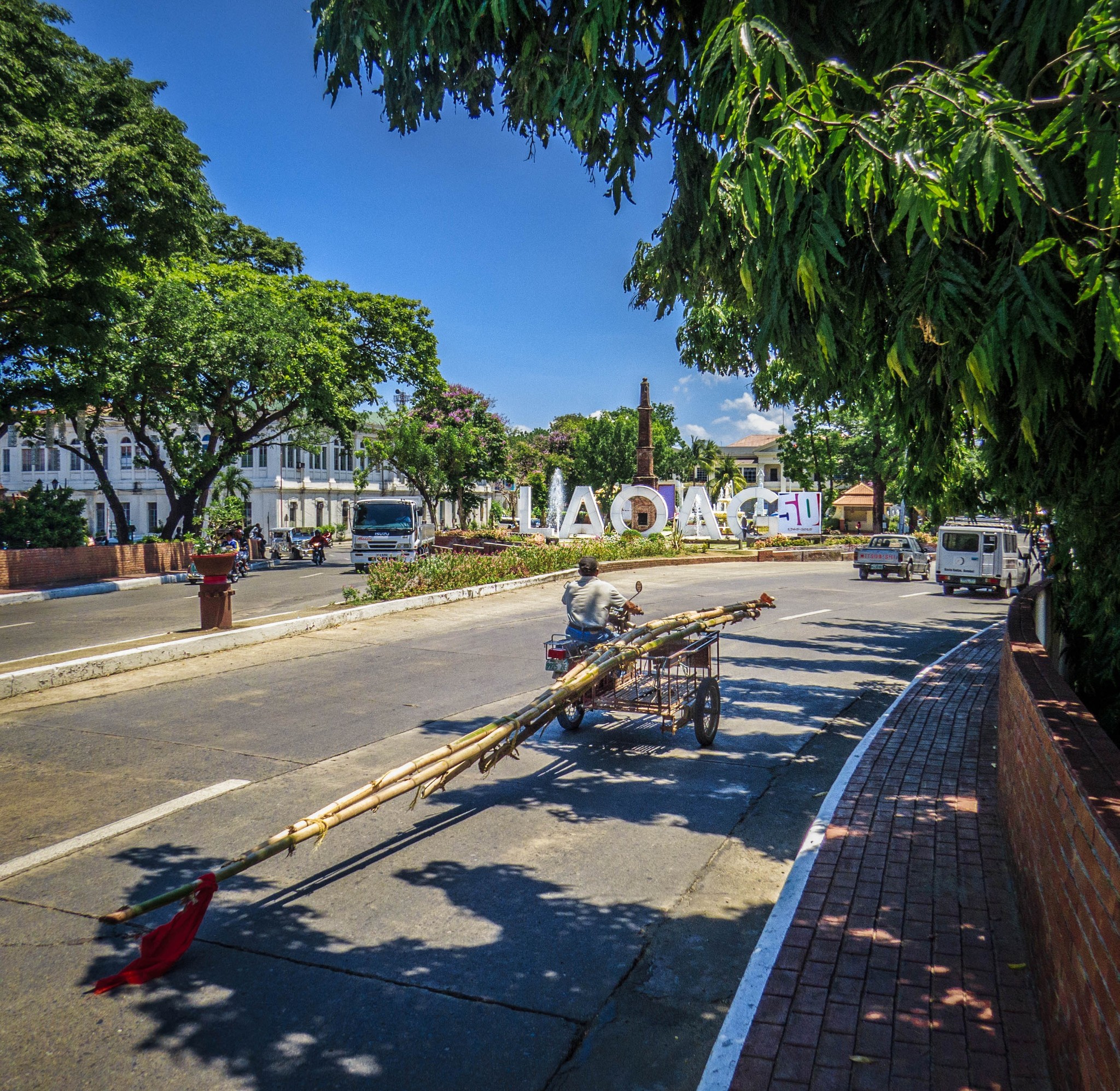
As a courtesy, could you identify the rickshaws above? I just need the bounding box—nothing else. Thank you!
[542,581,720,746]
[186,529,248,585]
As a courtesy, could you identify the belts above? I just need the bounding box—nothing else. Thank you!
[569,622,602,632]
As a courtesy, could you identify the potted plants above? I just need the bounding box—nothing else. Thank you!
[183,496,248,576]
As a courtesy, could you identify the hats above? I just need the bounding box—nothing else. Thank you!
[258,527,263,529]
[579,557,599,574]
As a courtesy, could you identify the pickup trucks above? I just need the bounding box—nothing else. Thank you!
[853,534,932,582]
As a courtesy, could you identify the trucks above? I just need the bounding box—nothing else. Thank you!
[268,526,316,560]
[935,515,1031,599]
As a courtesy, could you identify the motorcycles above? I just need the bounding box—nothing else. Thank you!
[311,542,326,566]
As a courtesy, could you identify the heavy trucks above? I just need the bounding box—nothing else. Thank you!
[348,496,436,572]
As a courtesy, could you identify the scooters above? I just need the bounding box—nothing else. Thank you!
[1036,537,1050,564]
[326,537,334,549]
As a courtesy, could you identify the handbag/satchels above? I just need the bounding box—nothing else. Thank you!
[262,539,267,545]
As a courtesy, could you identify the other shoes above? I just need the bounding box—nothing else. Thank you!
[324,558,326,560]
[244,565,250,570]
[259,548,263,551]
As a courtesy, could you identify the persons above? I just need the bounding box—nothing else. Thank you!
[221,524,267,576]
[844,519,848,531]
[562,557,642,680]
[741,517,762,542]
[884,540,890,546]
[857,521,861,535]
[309,530,331,562]
[1041,528,1056,580]
[175,527,183,540]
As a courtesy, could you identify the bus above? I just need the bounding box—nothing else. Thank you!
[497,517,541,529]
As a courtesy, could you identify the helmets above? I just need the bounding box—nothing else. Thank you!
[315,529,321,533]
[235,529,244,538]
[326,530,330,533]
